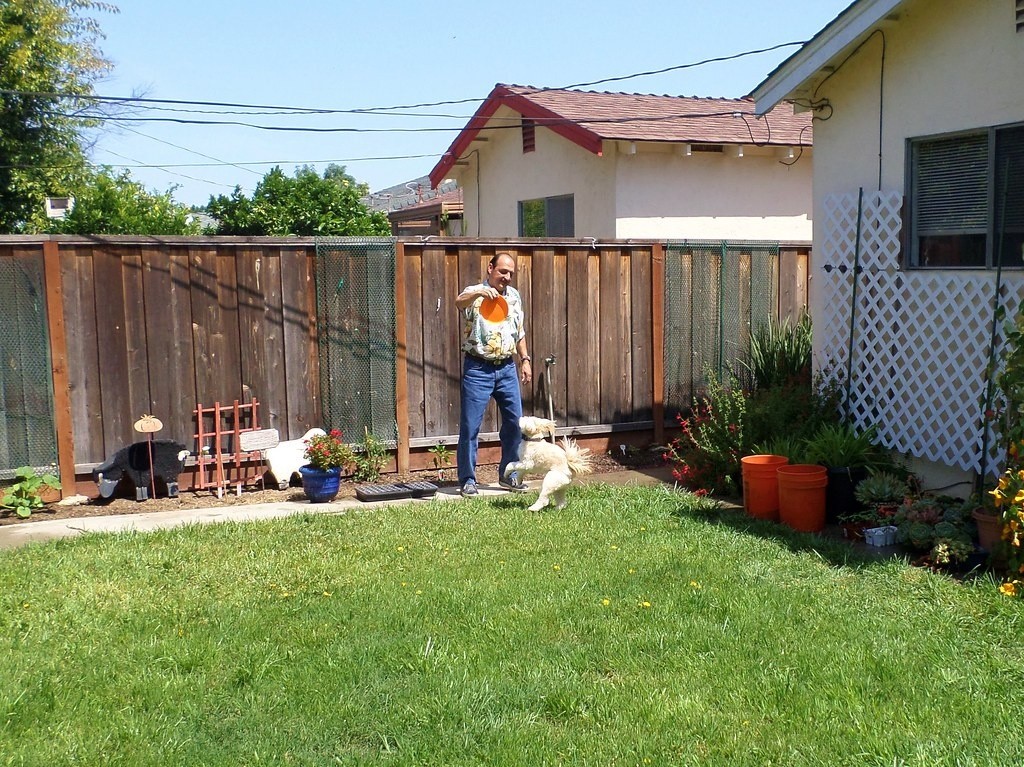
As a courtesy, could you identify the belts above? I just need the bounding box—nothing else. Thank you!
[465,353,513,366]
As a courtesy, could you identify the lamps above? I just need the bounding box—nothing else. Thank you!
[789,147,795,157]
[631,141,637,155]
[686,144,692,156]
[738,144,744,157]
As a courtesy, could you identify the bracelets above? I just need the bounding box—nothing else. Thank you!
[521,356,531,362]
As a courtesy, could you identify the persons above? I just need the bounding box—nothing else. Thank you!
[454,253,531,497]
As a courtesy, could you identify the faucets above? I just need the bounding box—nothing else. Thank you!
[544,353,557,367]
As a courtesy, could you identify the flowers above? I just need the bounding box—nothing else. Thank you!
[303,429,352,471]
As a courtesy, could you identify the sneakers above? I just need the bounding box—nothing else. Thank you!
[498,476,530,490]
[459,483,479,497]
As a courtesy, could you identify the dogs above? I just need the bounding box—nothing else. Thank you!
[503,416,593,512]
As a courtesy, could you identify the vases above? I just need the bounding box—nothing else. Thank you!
[300,465,342,501]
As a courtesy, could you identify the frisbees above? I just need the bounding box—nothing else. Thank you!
[480,295,508,323]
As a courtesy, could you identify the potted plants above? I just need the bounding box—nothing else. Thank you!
[845,467,1008,566]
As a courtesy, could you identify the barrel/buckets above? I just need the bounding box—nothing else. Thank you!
[741,454,789,519]
[777,464,829,531]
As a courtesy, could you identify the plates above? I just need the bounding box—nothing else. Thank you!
[481,296,508,323]
